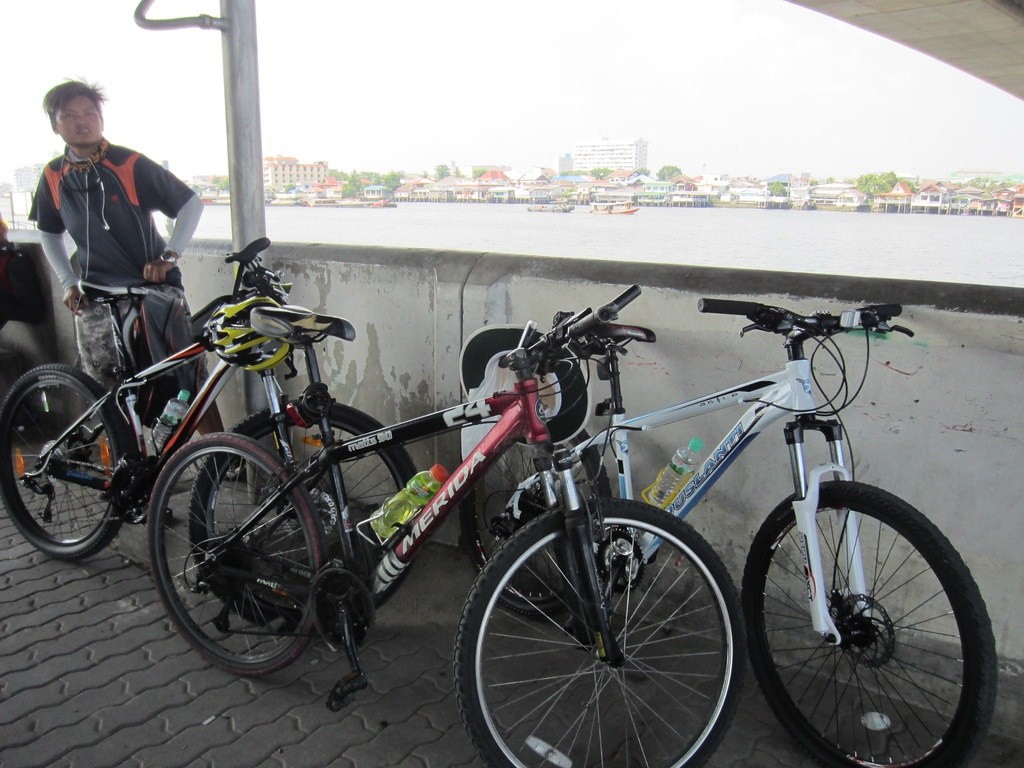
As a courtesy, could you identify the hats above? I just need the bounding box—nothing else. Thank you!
[458,323,591,446]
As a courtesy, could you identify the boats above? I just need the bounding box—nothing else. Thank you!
[526,205,574,212]
[584,202,640,215]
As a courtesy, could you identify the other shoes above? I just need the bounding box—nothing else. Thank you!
[123,494,173,524]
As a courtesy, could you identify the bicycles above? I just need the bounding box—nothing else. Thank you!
[187,295,998,766]
[1,239,423,635]
[146,281,747,768]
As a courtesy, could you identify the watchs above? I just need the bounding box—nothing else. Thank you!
[160,252,176,267]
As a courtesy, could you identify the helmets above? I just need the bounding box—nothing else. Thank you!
[202,296,293,372]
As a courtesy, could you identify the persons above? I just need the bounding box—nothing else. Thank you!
[26,81,289,500]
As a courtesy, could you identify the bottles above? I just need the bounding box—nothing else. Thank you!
[649,437,705,509]
[148,389,190,452]
[369,463,449,538]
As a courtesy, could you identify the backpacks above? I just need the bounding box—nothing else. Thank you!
[120,269,199,430]
